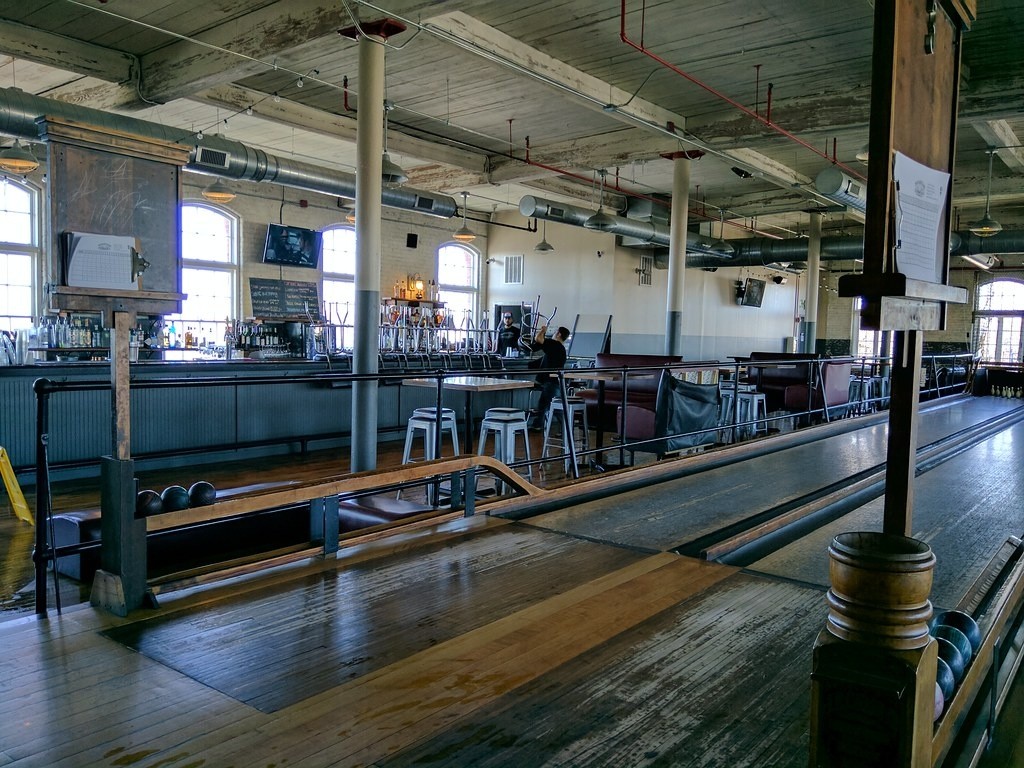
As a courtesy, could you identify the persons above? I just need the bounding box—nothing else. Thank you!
[496,311,521,357]
[530,326,570,438]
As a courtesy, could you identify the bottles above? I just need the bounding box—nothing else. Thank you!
[29,318,216,349]
[380,304,447,350]
[234,320,284,350]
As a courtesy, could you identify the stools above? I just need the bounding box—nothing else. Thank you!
[396,417,459,503]
[719,379,767,444]
[850,375,884,414]
[486,408,525,484]
[381,104,407,182]
[551,396,585,405]
[412,406,456,431]
[474,416,532,496]
[539,401,588,474]
[302,295,556,387]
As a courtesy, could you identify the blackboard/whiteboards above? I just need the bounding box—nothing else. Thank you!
[262,223,322,268]
[249,277,320,321]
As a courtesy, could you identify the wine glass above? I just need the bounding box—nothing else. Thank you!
[260,342,291,359]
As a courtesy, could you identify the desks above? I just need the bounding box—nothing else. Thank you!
[548,372,654,474]
[744,364,796,436]
[860,363,890,378]
[401,376,534,507]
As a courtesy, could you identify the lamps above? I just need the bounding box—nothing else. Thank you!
[968,148,1004,237]
[786,222,807,269]
[409,271,423,295]
[791,181,814,187]
[601,67,667,112]
[584,170,617,232]
[346,209,355,221]
[705,210,733,254]
[0,56,40,174]
[532,221,554,254]
[201,106,236,204]
[452,194,475,240]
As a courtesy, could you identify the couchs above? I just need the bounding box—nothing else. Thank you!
[783,355,852,424]
[920,366,967,401]
[738,352,822,413]
[574,353,683,437]
[617,360,719,451]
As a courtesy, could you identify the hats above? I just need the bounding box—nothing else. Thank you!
[504,312,512,318]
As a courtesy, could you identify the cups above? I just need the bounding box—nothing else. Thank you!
[15,329,34,366]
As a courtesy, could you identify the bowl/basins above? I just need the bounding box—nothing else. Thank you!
[56,355,79,362]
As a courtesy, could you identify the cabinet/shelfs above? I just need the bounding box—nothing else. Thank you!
[380,298,449,353]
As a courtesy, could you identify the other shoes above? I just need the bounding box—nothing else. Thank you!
[531,422,540,430]
